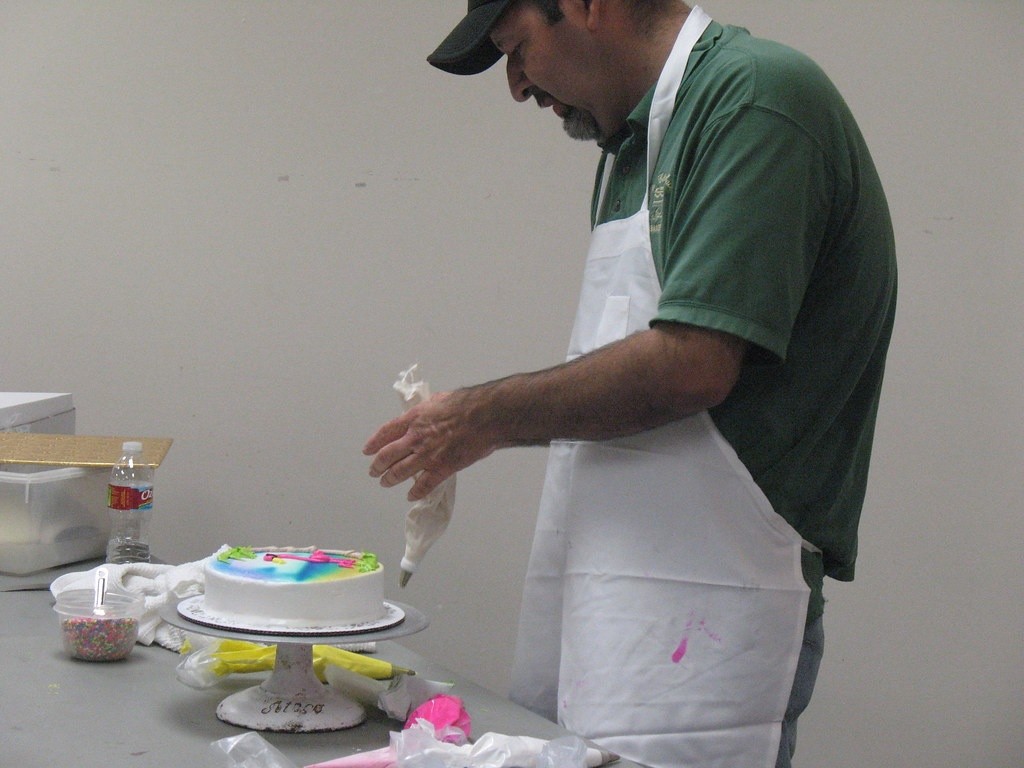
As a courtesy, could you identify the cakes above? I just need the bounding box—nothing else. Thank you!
[199,545,384,628]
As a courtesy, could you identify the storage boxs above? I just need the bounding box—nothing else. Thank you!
[0,468,120,576]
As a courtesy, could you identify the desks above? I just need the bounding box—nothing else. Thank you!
[0,554,651,767]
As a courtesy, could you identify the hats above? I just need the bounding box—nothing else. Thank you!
[426,0,518,75]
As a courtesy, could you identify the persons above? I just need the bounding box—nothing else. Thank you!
[364,1,896,768]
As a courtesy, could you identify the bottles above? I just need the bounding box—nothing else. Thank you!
[105,441,152,565]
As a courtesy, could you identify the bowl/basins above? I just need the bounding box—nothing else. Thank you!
[52,589,147,662]
